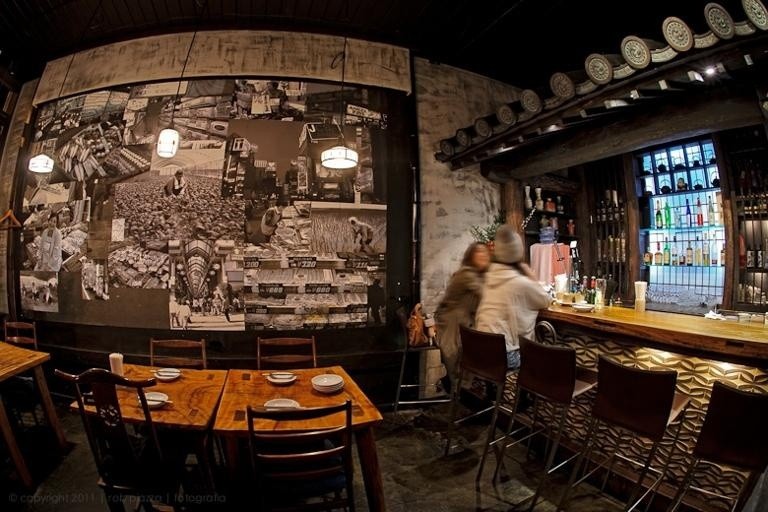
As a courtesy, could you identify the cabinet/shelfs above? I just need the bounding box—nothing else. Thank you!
[719,124,768,317]
[525,185,580,241]
[585,155,629,299]
[637,134,724,269]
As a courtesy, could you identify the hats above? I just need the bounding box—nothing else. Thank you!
[492,224,525,264]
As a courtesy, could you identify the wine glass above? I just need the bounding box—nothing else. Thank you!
[647,285,717,314]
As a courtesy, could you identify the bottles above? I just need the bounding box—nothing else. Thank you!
[738,280,766,305]
[539,215,550,229]
[697,197,703,226]
[540,271,596,304]
[738,233,768,269]
[567,218,576,236]
[524,186,564,215]
[737,161,768,218]
[590,196,627,263]
[675,207,682,229]
[550,216,558,234]
[684,199,692,227]
[643,232,726,267]
[708,195,715,226]
[664,202,672,229]
[655,200,663,229]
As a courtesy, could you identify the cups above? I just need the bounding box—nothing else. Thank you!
[108,353,124,378]
[595,278,607,310]
[634,281,647,312]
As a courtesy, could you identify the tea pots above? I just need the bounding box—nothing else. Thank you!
[641,150,720,197]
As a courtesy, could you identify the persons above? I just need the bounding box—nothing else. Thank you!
[349,216,375,253]
[261,197,289,243]
[164,168,188,202]
[169,289,246,331]
[21,281,29,296]
[44,283,58,305]
[471,223,552,416]
[34,212,65,272]
[30,282,41,304]
[434,239,490,396]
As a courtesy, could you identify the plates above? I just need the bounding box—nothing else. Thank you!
[153,367,181,381]
[267,371,298,384]
[310,373,345,394]
[571,304,596,312]
[264,398,300,413]
[135,391,169,409]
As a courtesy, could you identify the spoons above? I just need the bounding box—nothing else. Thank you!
[255,404,297,411]
[259,371,301,377]
[148,369,186,375]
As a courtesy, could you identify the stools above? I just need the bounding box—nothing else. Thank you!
[493,334,599,510]
[445,324,537,482]
[393,307,460,417]
[670,381,767,510]
[556,354,691,510]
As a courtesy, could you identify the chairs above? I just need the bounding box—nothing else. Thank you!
[4,320,39,426]
[246,399,356,512]
[150,338,215,465]
[54,367,191,512]
[257,335,316,370]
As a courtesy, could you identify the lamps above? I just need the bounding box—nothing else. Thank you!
[27,53,75,173]
[321,36,358,169]
[156,31,197,158]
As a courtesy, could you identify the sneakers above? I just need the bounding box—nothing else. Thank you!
[436,376,452,394]
[459,437,480,453]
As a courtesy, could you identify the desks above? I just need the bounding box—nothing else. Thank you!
[459,307,766,510]
